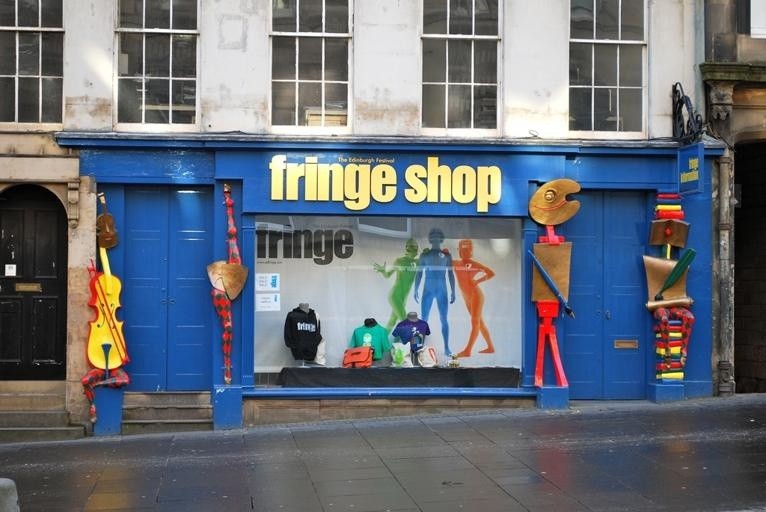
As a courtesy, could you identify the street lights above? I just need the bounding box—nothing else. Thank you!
[390,342,414,368]
[341,346,374,369]
[415,345,439,368]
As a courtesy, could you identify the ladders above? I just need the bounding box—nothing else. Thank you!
[96,192,119,249]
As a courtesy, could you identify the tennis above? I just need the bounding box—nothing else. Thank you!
[89,247,128,372]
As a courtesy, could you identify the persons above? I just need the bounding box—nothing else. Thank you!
[349,317,392,364]
[283,301,322,363]
[413,227,457,359]
[393,314,432,367]
[442,238,495,358]
[373,236,429,366]
